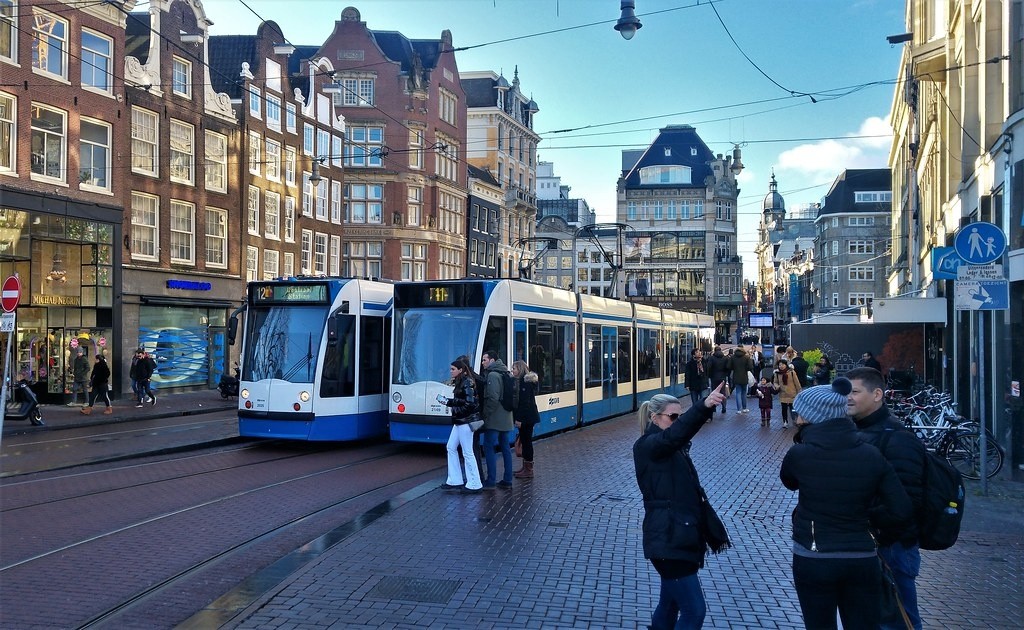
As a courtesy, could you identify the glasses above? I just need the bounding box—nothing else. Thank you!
[138,355,142,357]
[648,413,683,421]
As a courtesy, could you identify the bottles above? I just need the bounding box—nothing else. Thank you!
[944,502,958,515]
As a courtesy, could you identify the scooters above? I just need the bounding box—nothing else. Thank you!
[217,362,241,400]
[0,370,45,426]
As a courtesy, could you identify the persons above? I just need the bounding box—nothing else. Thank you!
[456,356,485,485]
[775,346,834,388]
[756,377,781,427]
[618,351,629,378]
[632,380,732,630]
[440,360,483,493]
[129,348,157,407]
[863,352,881,372]
[707,345,765,414]
[643,350,656,379]
[324,358,346,385]
[67,350,90,407]
[773,359,803,428]
[482,350,515,490]
[511,361,541,477]
[80,354,113,414]
[780,377,913,630]
[530,345,547,392]
[845,367,922,630]
[684,349,714,423]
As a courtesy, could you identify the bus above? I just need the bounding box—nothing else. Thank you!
[389,223,715,449]
[225,274,392,443]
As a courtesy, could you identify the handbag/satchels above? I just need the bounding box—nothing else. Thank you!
[877,554,900,624]
[747,370,757,387]
[469,419,485,431]
[514,433,522,457]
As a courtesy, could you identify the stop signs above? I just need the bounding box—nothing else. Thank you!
[1,276,22,313]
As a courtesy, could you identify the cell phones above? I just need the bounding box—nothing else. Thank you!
[436,394,448,404]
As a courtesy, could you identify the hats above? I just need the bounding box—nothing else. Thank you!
[76,347,83,353]
[792,377,852,424]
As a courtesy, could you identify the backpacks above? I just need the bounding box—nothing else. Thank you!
[488,370,514,411]
[880,428,965,551]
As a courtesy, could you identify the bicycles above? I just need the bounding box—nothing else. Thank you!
[889,387,1006,478]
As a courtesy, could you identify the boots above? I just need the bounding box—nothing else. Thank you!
[79,406,93,415]
[103,405,113,415]
[512,458,525,475]
[515,461,534,477]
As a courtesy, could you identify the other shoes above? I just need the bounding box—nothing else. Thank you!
[737,410,741,414]
[767,419,770,427]
[482,480,496,490]
[81,403,88,407]
[782,422,789,429]
[706,418,711,423]
[457,486,483,495]
[66,402,76,406]
[441,483,464,491]
[743,409,749,413]
[761,421,765,426]
[496,480,512,488]
[722,409,726,413]
[132,392,157,408]
